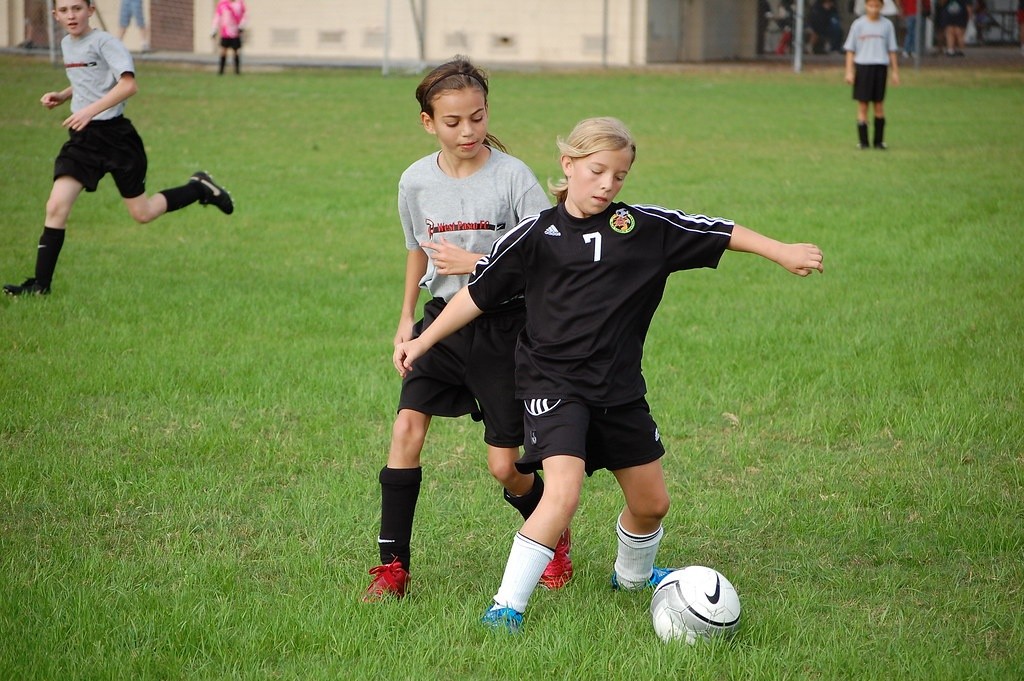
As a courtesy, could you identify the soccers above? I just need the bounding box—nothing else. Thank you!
[650,565,744,645]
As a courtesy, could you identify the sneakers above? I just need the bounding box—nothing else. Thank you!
[611,564,676,591]
[482,599,524,632]
[189,170,235,214]
[361,555,412,601]
[541,528,574,589]
[3,277,51,296]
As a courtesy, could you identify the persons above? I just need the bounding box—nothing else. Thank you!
[1016,0,1024,57]
[358,59,571,604]
[4,0,235,297]
[211,0,250,75]
[395,116,822,632]
[844,0,898,149]
[758,0,989,58]
[115,0,152,53]
